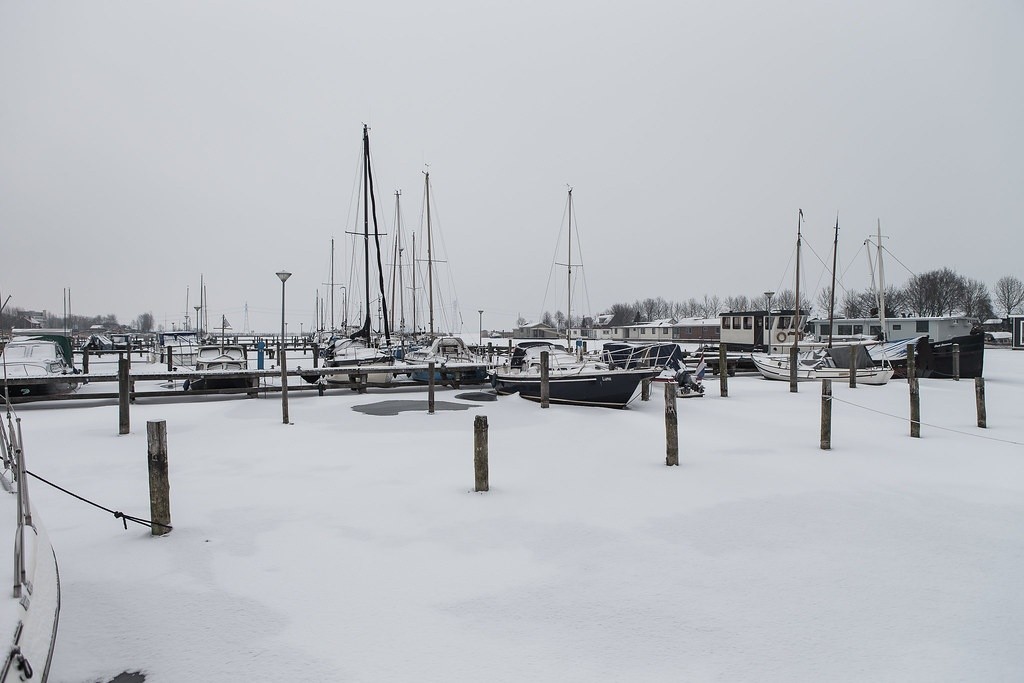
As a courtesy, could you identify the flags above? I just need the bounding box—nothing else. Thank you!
[695,356,705,378]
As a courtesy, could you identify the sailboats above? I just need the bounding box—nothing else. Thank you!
[172,122,984,412]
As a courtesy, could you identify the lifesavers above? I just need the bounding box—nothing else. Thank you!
[777,331,787,342]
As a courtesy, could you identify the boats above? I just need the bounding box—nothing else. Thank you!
[0,338,79,405]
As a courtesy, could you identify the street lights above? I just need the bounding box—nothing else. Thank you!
[194,306,202,345]
[275,270,293,350]
[478,310,484,346]
[763,291,775,355]
